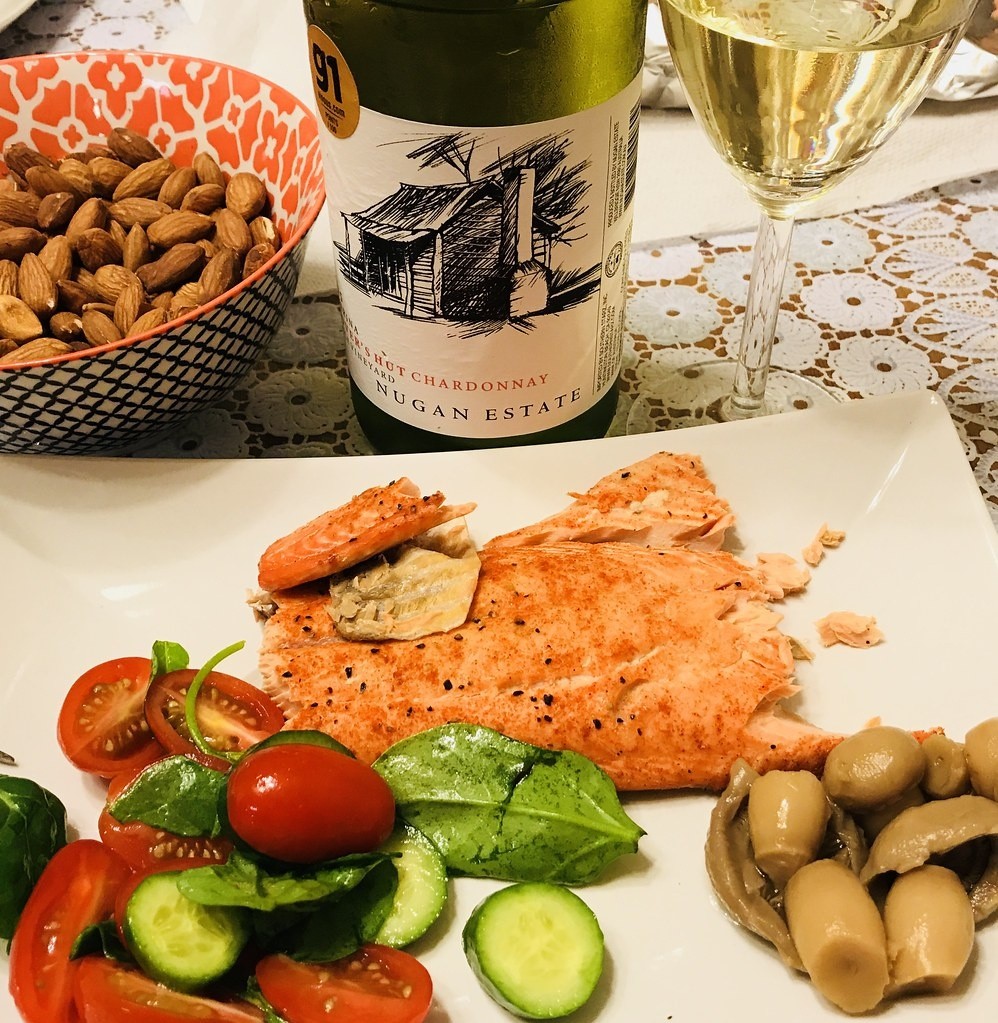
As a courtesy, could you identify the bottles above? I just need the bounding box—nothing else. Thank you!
[301,0,632,447]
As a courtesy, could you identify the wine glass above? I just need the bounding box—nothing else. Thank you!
[624,0,976,437]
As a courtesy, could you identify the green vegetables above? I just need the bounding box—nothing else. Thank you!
[0,635,648,1023]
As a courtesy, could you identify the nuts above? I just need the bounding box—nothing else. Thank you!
[0,126,280,368]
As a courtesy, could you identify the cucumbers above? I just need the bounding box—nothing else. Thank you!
[364,819,448,950]
[462,880,606,1020]
[121,869,252,993]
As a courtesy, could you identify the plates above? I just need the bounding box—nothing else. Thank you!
[0,388,998,1023]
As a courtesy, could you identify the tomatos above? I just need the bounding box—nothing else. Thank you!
[115,868,185,940]
[227,744,395,863]
[9,839,132,1023]
[58,656,163,777]
[98,753,234,866]
[256,941,433,1023]
[143,669,284,757]
[70,952,264,1023]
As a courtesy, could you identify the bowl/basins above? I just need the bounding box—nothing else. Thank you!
[0,49,326,455]
